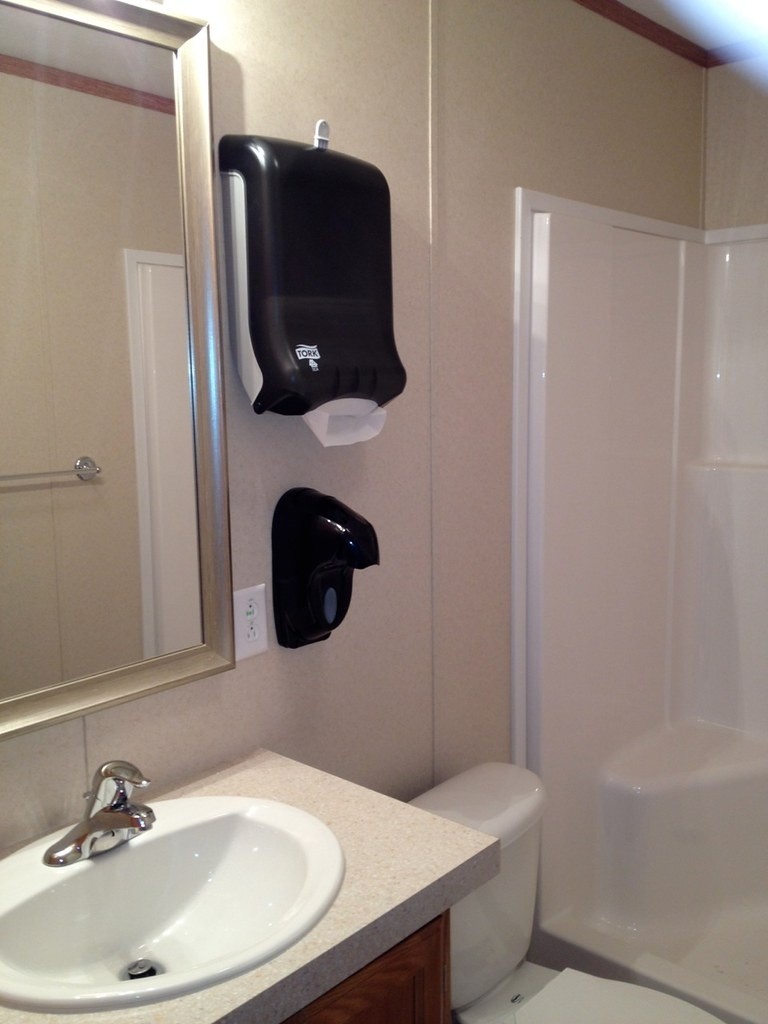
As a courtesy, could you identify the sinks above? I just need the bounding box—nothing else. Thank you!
[0,791,345,1013]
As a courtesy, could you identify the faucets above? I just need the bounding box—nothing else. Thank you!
[44,758,157,869]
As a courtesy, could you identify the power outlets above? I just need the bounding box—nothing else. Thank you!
[233,583,270,665]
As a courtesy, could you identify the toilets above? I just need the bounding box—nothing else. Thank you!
[399,756,714,1023]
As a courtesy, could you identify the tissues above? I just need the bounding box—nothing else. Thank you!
[220,120,409,451]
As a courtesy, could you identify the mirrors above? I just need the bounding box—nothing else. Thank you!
[0,1,234,740]
